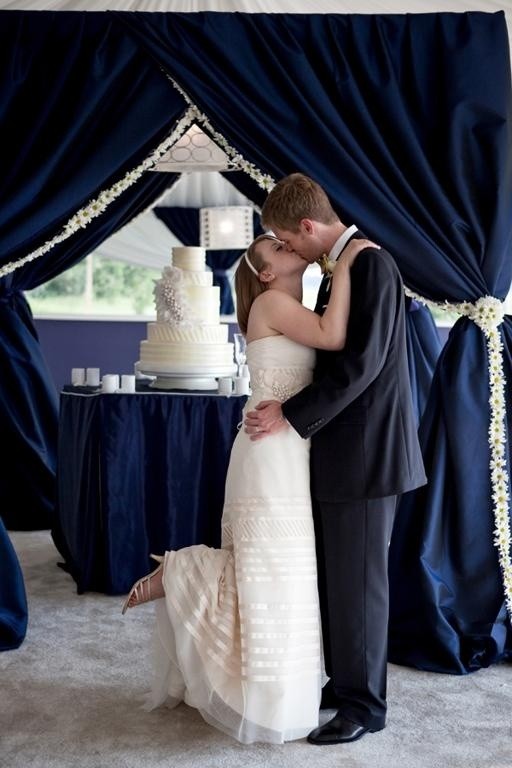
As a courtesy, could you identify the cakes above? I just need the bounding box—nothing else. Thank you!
[139,247,235,370]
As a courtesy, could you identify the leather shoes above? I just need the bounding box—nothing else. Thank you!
[308,714,382,746]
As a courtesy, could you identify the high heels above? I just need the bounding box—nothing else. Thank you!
[122,553,164,613]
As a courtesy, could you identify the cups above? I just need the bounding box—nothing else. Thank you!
[217,377,250,397]
[71,368,136,394]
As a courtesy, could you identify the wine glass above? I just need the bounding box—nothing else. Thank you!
[233,334,248,376]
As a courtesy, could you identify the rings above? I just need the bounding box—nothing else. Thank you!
[255,426,260,436]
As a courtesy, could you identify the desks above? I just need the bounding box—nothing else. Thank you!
[55,379,246,595]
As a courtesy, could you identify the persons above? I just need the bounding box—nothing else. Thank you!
[121,233,382,748]
[243,172,429,744]
